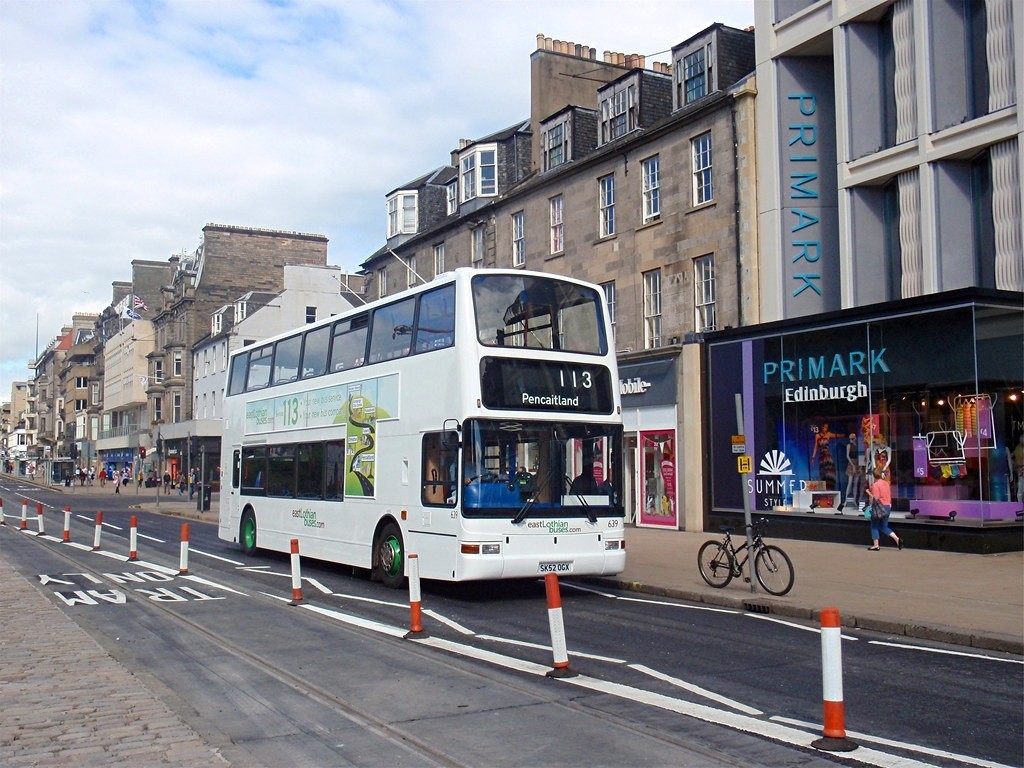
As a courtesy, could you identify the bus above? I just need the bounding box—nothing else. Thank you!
[217,267,629,591]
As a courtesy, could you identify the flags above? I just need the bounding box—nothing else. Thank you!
[133,296,147,310]
[122,308,143,320]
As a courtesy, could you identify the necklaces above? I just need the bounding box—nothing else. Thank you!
[880,447,885,449]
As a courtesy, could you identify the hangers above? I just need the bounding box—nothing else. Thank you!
[964,398,969,404]
[970,398,976,404]
[957,398,963,408]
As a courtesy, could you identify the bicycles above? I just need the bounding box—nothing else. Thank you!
[697,517,795,596]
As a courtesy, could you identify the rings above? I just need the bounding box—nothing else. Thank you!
[468,481,469,482]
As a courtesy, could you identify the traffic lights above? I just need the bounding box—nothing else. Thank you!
[193,436,201,457]
[141,448,146,459]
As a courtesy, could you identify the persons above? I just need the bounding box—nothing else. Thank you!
[597,467,613,502]
[515,467,533,483]
[10,465,12,473]
[866,468,903,551]
[189,467,200,500]
[28,464,35,481]
[177,471,185,495]
[76,465,95,486]
[450,445,488,498]
[843,433,891,507]
[99,468,143,495]
[811,423,846,489]
[42,466,45,483]
[163,471,171,494]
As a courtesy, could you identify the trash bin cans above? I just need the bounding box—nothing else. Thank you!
[197,485,212,512]
[65,475,71,486]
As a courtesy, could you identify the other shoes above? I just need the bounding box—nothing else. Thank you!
[898,539,903,550]
[868,547,880,551]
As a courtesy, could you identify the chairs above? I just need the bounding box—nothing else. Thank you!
[252,364,344,391]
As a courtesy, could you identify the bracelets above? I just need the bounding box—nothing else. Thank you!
[812,457,814,460]
[882,471,885,473]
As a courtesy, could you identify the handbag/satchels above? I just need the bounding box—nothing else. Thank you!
[113,481,117,485]
[176,483,180,488]
[864,497,889,521]
